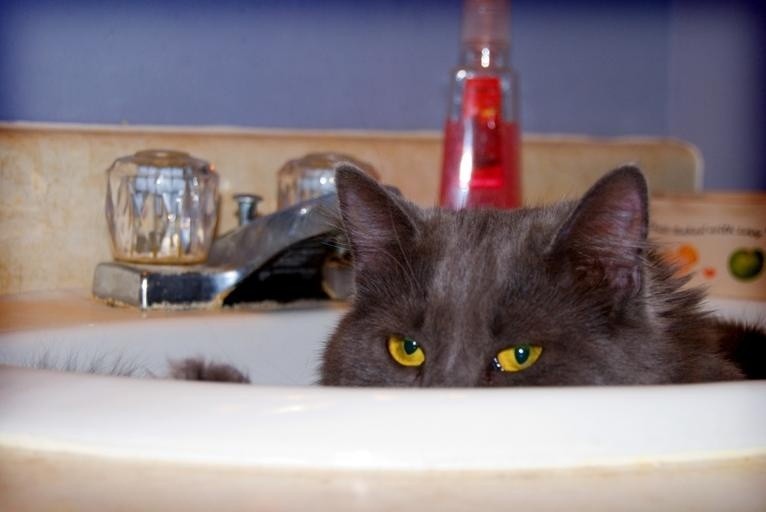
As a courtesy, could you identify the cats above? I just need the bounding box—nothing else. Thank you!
[26,161,765,389]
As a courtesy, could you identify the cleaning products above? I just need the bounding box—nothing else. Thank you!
[438,0,523,213]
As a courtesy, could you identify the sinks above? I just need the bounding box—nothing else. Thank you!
[0,297,766,473]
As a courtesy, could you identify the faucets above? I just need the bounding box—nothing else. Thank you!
[212,183,401,270]
